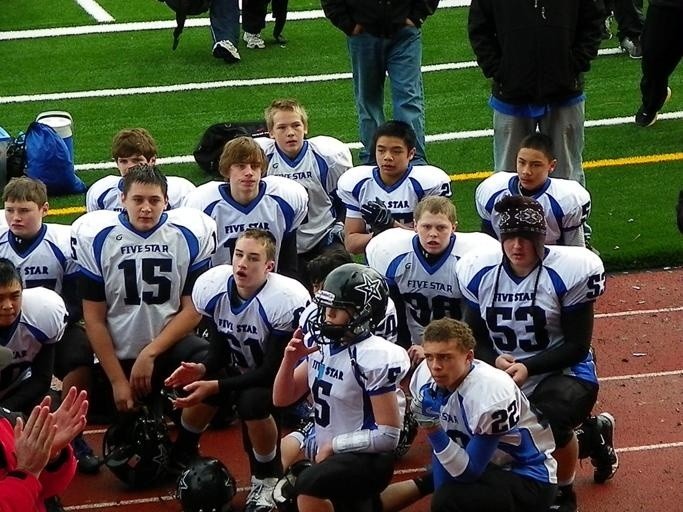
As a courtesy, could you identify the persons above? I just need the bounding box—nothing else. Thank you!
[318,0,440,166]
[635,0,683,128]
[163,226,311,512]
[474,132,603,256]
[453,196,619,512]
[208,0,270,62]
[0,175,81,324]
[468,0,602,189]
[0,385,92,511]
[335,119,452,257]
[183,136,310,275]
[307,248,420,460]
[262,96,355,264]
[272,262,433,512]
[0,257,70,413]
[85,126,196,213]
[365,195,500,369]
[408,316,560,511]
[59,163,218,461]
[610,0,646,60]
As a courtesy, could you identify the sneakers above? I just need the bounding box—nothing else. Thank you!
[619,36,643,59]
[635,86,671,127]
[76,447,103,474]
[549,478,578,512]
[242,473,277,512]
[242,31,265,49]
[585,412,619,483]
[212,39,241,63]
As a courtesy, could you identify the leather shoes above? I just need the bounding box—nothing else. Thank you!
[273,31,289,44]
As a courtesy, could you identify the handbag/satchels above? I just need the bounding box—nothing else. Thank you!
[193,121,268,177]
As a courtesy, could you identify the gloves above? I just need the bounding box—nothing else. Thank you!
[358,197,394,235]
[409,386,443,427]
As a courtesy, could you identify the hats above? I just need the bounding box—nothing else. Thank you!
[494,194,548,266]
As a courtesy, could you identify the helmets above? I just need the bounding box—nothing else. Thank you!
[102,410,168,485]
[174,456,237,512]
[307,263,389,345]
